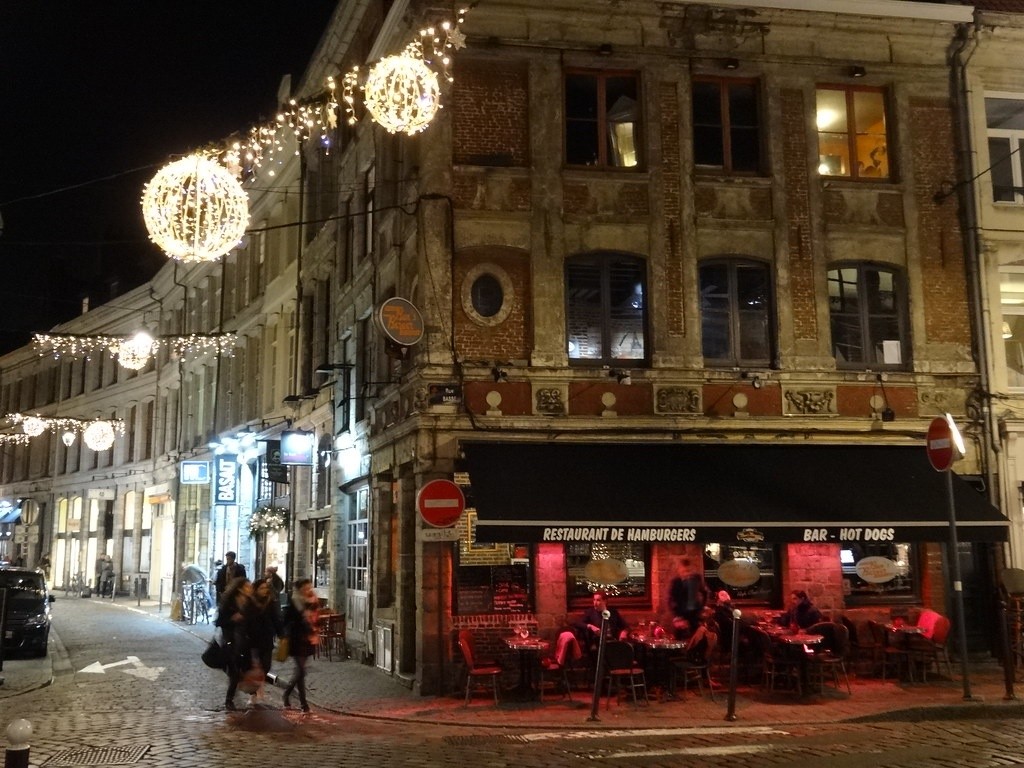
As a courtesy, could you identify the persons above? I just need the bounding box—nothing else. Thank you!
[773,590,830,641]
[667,557,763,686]
[212,551,321,712]
[95,553,114,597]
[574,591,645,670]
[38,553,51,574]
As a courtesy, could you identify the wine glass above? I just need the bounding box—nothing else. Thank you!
[520,629,529,644]
[514,624,521,641]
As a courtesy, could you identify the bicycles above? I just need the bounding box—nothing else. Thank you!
[105,574,113,598]
[182,582,212,625]
[72,571,84,596]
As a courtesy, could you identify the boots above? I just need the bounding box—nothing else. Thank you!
[283,670,310,711]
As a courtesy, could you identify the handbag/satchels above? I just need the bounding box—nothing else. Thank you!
[201,637,232,669]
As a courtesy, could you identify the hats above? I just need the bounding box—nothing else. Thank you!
[268,563,279,573]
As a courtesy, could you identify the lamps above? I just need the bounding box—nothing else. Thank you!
[492,367,508,384]
[315,364,355,374]
[850,66,866,77]
[598,43,612,56]
[305,380,338,396]
[728,59,739,70]
[282,395,315,402]
[609,370,631,386]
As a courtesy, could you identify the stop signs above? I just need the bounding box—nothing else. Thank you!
[417,480,464,527]
[927,417,954,471]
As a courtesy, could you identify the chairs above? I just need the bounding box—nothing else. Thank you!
[533,632,573,702]
[582,629,718,711]
[842,608,955,685]
[458,630,505,708]
[313,613,348,662]
[713,613,854,697]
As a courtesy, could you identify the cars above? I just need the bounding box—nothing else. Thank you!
[0,565,55,658]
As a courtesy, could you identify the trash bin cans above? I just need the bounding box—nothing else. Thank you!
[170,599,183,620]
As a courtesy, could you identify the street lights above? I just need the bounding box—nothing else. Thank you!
[943,414,973,698]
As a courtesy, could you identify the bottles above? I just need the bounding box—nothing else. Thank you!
[763,612,772,629]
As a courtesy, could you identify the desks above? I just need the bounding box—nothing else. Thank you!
[503,638,549,700]
[869,620,927,681]
[631,634,689,701]
[767,628,824,694]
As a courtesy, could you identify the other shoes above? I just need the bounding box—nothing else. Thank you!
[225,699,236,710]
[247,694,257,705]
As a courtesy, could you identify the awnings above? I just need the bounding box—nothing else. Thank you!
[456,438,1013,544]
[0,507,22,524]
